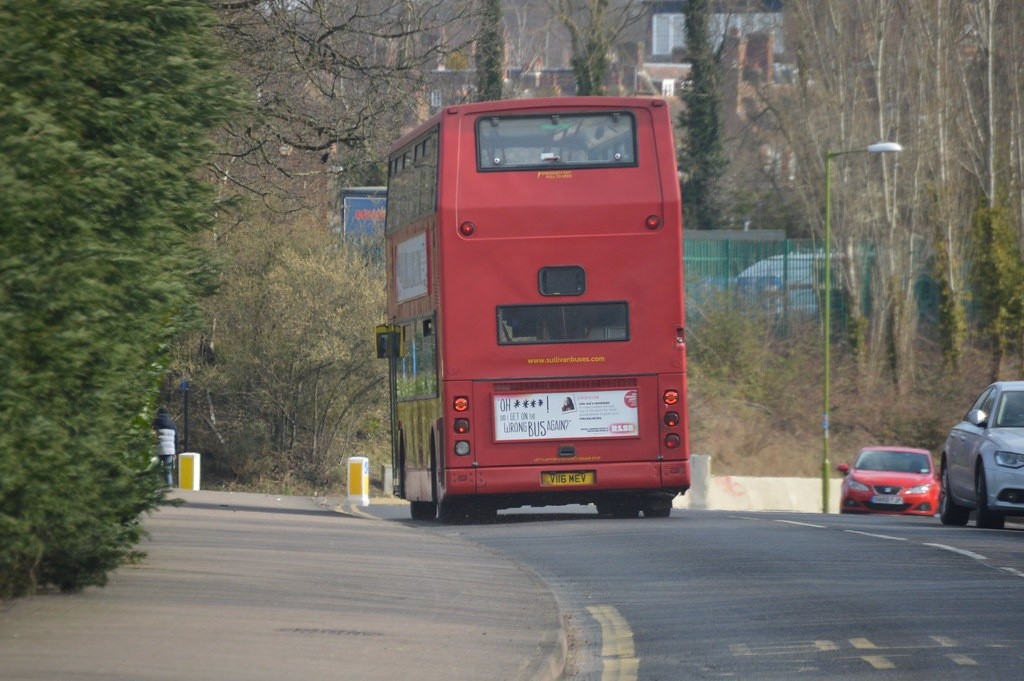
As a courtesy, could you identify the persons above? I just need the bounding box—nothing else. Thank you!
[151,406,178,488]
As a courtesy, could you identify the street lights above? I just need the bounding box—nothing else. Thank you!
[822,140,904,518]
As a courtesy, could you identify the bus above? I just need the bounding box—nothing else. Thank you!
[373,95,691,523]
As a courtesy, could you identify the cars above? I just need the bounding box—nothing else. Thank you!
[834,444,942,520]
[940,379,1024,529]
